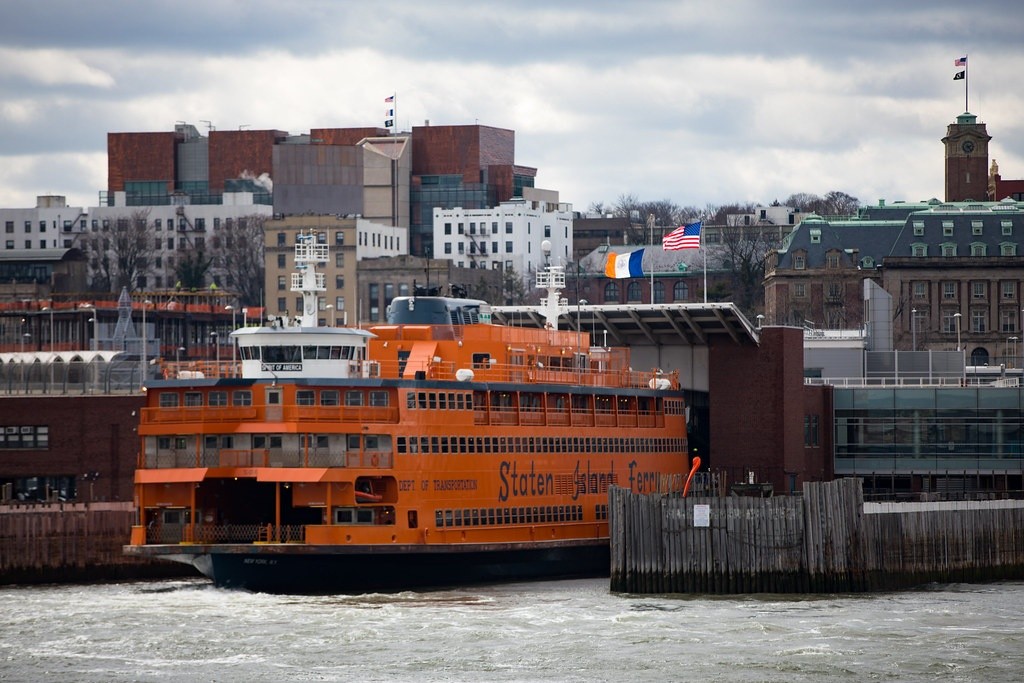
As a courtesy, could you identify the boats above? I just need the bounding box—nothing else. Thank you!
[120,295,693,596]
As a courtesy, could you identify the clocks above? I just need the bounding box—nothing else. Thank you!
[962,141,975,153]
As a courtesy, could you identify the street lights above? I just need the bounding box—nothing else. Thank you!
[756,315,764,327]
[953,313,962,351]
[326,304,334,327]
[242,307,248,327]
[225,304,237,377]
[540,240,552,266]
[603,329,608,348]
[176,346,186,380]
[88,318,98,389]
[85,302,95,310]
[911,309,916,350]
[22,332,32,395]
[210,331,220,378]
[42,305,54,397]
[1011,336,1018,368]
[142,300,154,381]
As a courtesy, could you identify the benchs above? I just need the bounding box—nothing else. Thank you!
[161,360,240,380]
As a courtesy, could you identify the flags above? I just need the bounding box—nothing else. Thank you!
[955,57,966,66]
[663,222,701,251]
[385,96,394,102]
[953,71,965,79]
[605,248,646,278]
[385,109,393,116]
[385,120,393,126]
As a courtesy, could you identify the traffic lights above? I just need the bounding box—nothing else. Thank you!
[82,468,100,502]
